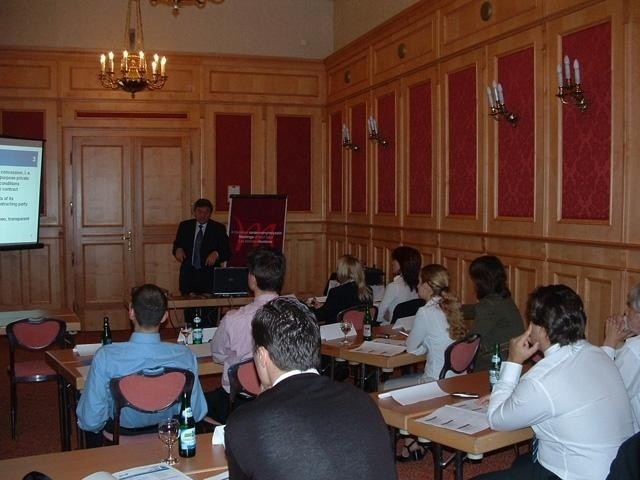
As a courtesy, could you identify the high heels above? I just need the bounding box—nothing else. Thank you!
[396,439,423,463]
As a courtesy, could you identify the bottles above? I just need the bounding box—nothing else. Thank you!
[176,390,197,458]
[191,307,203,344]
[487,342,502,390]
[363,305,373,340]
[102,317,112,346]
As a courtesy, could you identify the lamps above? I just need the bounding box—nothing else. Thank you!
[556,56,588,111]
[487,80,518,126]
[342,127,359,151]
[368,116,389,149]
[150,0,224,18]
[98,0,167,100]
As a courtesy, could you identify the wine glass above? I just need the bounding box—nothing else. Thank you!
[179,322,192,344]
[158,418,181,465]
[340,321,353,346]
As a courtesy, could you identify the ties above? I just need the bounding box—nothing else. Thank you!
[192,224,204,271]
[531,436,541,464]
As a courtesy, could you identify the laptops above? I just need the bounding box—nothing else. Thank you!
[212,267,250,296]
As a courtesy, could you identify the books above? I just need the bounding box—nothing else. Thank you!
[349,337,407,358]
[414,398,490,436]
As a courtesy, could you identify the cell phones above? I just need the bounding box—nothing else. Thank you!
[398,329,410,337]
[452,391,481,399]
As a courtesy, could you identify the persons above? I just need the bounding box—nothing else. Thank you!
[461,255,525,374]
[204,246,298,425]
[172,199,230,327]
[600,284,640,432]
[378,263,469,462]
[77,284,208,447]
[310,246,422,324]
[80,462,194,480]
[224,296,398,480]
[469,283,640,480]
